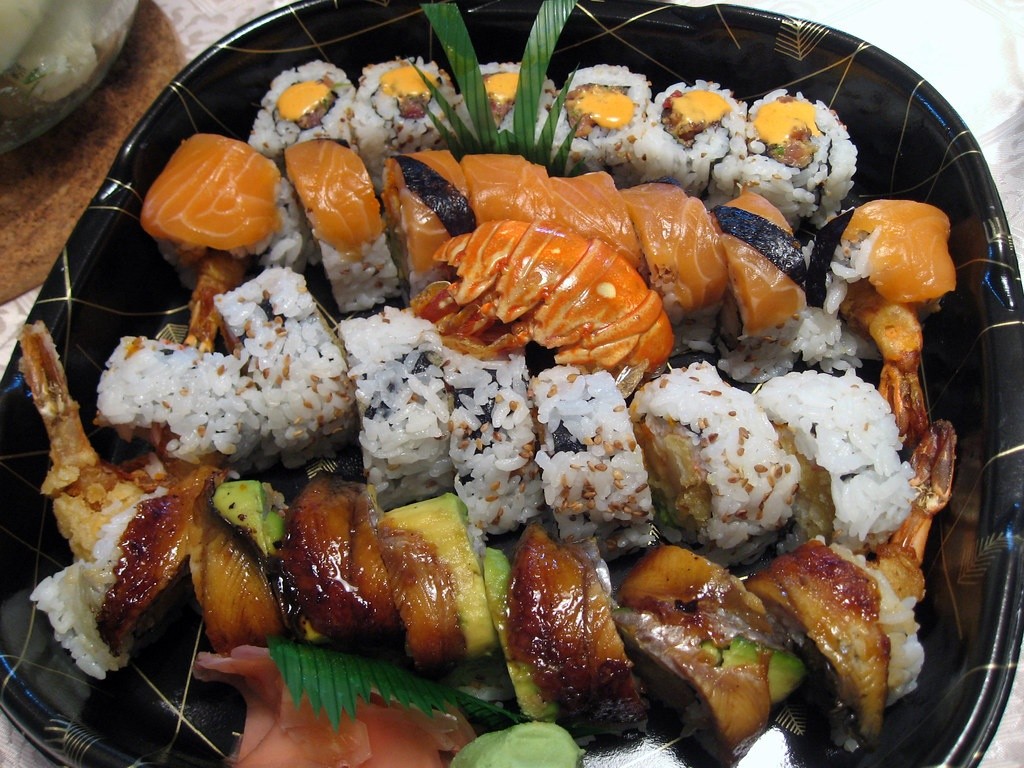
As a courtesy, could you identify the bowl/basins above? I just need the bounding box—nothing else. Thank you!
[0,1,1024,768]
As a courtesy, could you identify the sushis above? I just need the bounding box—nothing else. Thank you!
[11,53,955,756]
